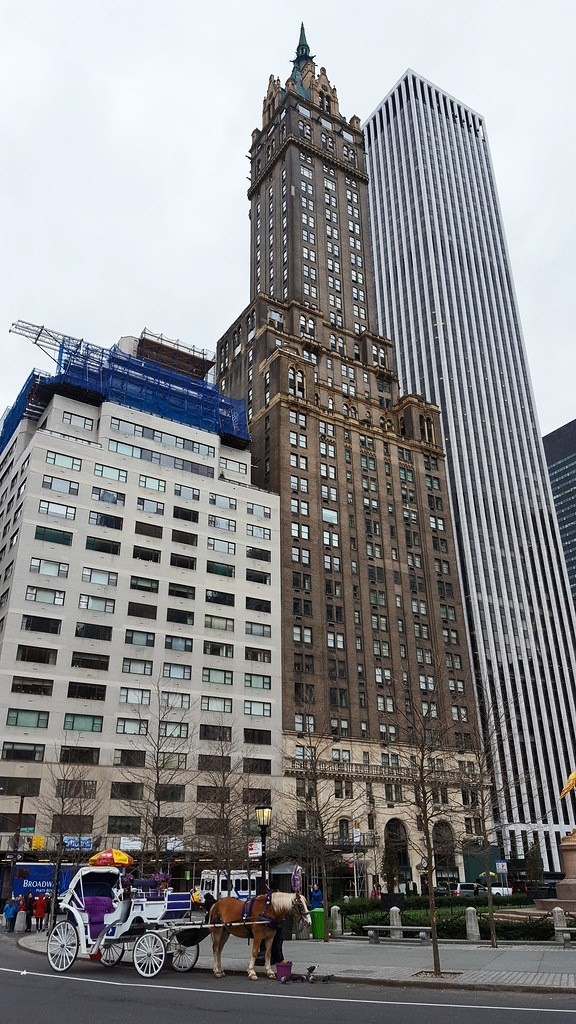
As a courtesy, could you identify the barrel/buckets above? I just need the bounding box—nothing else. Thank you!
[275,963,292,980]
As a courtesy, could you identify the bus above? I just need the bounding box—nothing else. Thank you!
[198,869,269,905]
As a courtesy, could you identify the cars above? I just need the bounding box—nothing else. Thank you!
[434,886,449,897]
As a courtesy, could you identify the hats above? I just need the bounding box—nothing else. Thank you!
[44,893,50,896]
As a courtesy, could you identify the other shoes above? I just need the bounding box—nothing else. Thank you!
[41,929,45,931]
[36,929,40,932]
[25,929,30,932]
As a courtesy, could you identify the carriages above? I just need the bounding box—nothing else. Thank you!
[45,866,313,979]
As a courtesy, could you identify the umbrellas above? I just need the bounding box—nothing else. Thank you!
[89,848,134,867]
[480,871,496,878]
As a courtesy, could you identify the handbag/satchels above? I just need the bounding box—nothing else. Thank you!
[2,912,6,926]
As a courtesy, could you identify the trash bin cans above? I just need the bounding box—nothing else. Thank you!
[310,908,327,939]
[295,915,310,939]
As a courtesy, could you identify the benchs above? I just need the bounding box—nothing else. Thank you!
[363,926,432,946]
[555,928,576,948]
[68,896,115,938]
[130,879,174,902]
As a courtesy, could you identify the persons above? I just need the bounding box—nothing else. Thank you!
[191,891,217,924]
[311,884,324,909]
[3,891,55,934]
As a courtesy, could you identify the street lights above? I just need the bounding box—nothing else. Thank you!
[254,795,273,895]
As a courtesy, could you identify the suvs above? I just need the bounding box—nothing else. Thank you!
[448,882,494,897]
[511,878,556,894]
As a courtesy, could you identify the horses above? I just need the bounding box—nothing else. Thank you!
[207,891,312,980]
